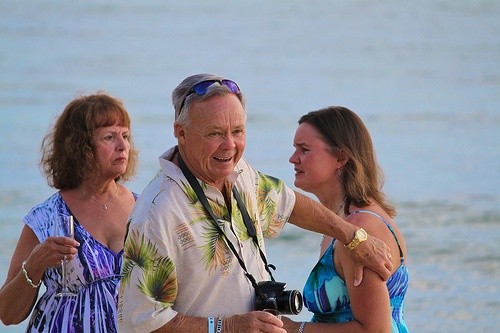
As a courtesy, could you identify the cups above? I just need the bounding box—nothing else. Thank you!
[264,308,281,320]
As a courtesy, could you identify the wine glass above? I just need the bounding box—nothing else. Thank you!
[52,215,77,297]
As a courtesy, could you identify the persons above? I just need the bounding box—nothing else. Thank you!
[117,74,393,333]
[0,88,140,333]
[280,105,409,333]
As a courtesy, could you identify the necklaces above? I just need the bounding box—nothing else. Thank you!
[322,201,344,237]
[83,185,119,210]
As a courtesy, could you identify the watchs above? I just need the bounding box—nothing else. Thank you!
[344,228,367,251]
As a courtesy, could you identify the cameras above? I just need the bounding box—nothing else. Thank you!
[254,280,303,316]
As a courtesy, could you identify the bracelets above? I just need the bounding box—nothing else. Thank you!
[22,261,42,288]
[208,315,223,333]
[298,322,307,333]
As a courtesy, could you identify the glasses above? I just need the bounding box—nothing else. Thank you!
[179,79,241,116]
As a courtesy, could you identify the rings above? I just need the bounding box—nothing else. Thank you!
[388,255,391,258]
